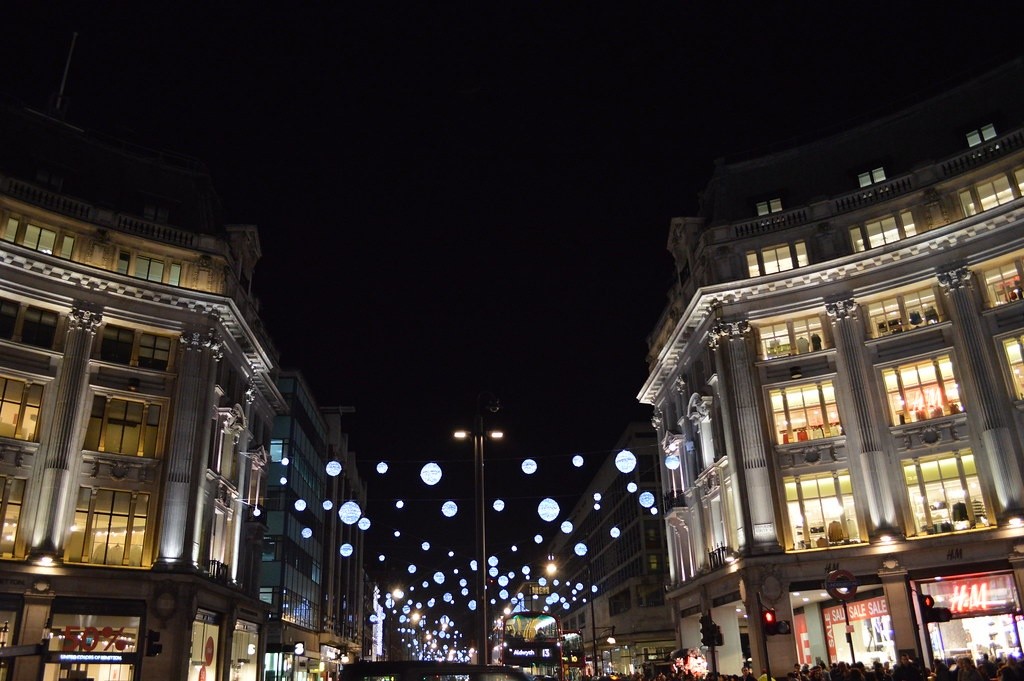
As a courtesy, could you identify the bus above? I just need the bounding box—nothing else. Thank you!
[498,610,565,681]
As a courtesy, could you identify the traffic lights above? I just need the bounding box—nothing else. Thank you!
[921,593,935,609]
[763,609,778,625]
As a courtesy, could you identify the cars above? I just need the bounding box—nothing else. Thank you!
[337,659,537,681]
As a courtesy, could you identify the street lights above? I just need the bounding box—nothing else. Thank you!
[591,626,617,676]
[452,424,504,665]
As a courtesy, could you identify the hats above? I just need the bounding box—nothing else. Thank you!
[762,667,767,674]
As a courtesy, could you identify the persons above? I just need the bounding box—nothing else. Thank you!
[577,650,1024,681]
[505,627,548,646]
